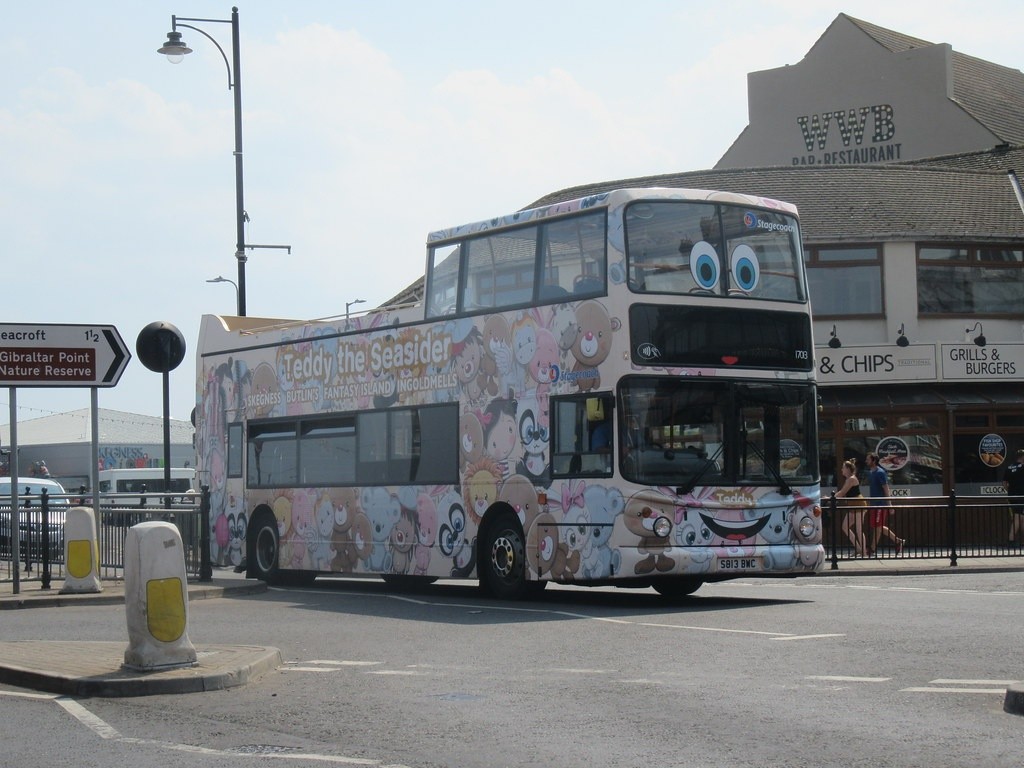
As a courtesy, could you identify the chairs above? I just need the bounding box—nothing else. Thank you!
[543,273,640,299]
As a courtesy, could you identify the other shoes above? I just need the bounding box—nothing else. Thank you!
[867,550,878,556]
[856,554,867,557]
[850,553,858,556]
[895,539,905,554]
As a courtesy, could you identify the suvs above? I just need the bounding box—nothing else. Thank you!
[0,476,71,554]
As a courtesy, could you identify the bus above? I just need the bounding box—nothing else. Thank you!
[193,186,826,603]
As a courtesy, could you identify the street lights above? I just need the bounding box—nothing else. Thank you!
[205,278,239,314]
[158,7,245,318]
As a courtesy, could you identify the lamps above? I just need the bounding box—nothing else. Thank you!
[896,323,909,347]
[828,325,842,348]
[966,322,987,347]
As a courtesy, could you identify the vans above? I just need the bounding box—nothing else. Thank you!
[96,466,197,523]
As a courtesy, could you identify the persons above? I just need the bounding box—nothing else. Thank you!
[1002,449,1024,550]
[865,452,907,557]
[822,458,868,558]
[591,403,647,455]
